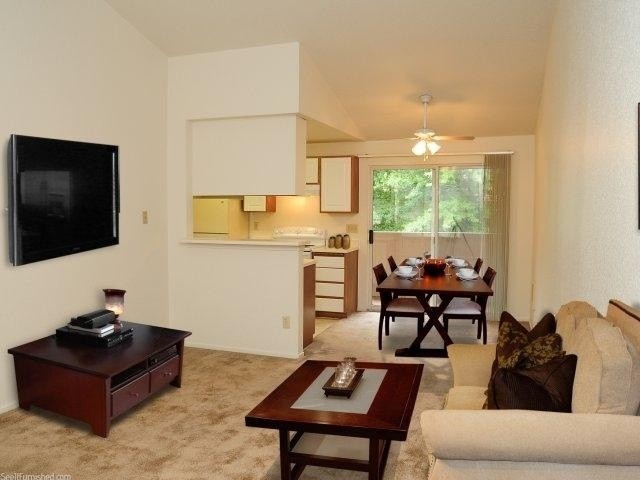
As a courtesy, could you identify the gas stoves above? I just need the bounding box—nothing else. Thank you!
[273,239,324,251]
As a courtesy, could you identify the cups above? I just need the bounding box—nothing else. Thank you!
[335,356,356,387]
[453,259,466,265]
[398,265,414,274]
[408,257,417,264]
[461,268,475,276]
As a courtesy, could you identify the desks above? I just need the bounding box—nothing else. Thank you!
[8,320,192,437]
[246,360,424,480]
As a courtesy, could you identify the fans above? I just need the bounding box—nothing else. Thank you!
[377,94,475,141]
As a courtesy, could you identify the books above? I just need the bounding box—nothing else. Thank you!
[70,322,115,334]
[70,328,116,339]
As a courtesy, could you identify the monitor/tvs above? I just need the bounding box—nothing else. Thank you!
[6,133,120,266]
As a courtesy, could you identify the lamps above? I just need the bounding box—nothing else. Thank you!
[412,137,441,161]
[103,289,126,323]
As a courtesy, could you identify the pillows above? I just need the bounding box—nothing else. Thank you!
[482,301,640,414]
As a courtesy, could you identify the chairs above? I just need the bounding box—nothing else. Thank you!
[420,299,640,480]
[373,255,496,351]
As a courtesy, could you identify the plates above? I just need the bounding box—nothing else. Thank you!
[405,260,420,266]
[456,272,478,280]
[395,270,417,278]
[451,263,469,268]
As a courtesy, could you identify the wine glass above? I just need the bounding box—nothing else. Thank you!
[415,251,452,280]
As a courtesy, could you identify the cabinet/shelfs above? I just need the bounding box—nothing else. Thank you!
[243,155,358,319]
